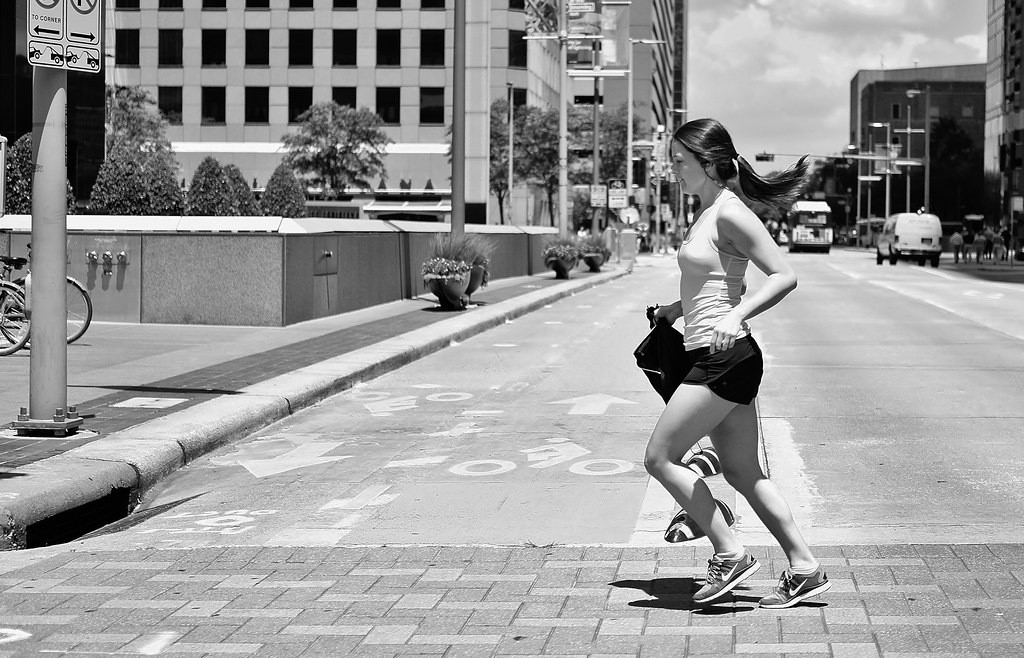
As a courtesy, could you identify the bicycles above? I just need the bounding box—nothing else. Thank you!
[0,254,32,357]
[0,242,93,351]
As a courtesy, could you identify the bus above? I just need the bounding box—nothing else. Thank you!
[785,199,834,255]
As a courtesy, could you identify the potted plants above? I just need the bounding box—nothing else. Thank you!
[421,231,500,308]
[576,231,612,273]
[540,237,582,280]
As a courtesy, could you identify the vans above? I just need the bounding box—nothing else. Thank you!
[875,213,944,268]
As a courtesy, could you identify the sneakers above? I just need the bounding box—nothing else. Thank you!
[692,546,761,603]
[758,563,830,609]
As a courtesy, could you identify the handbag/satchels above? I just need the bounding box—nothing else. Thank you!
[633,304,693,405]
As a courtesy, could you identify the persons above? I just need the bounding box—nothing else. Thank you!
[950,225,1011,265]
[641,116,833,608]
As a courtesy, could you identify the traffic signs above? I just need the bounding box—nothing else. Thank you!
[26,0,102,74]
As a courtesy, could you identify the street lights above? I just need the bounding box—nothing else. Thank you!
[626,36,669,227]
[846,144,862,247]
[654,107,690,253]
[505,82,516,226]
[866,121,893,222]
[905,85,931,213]
